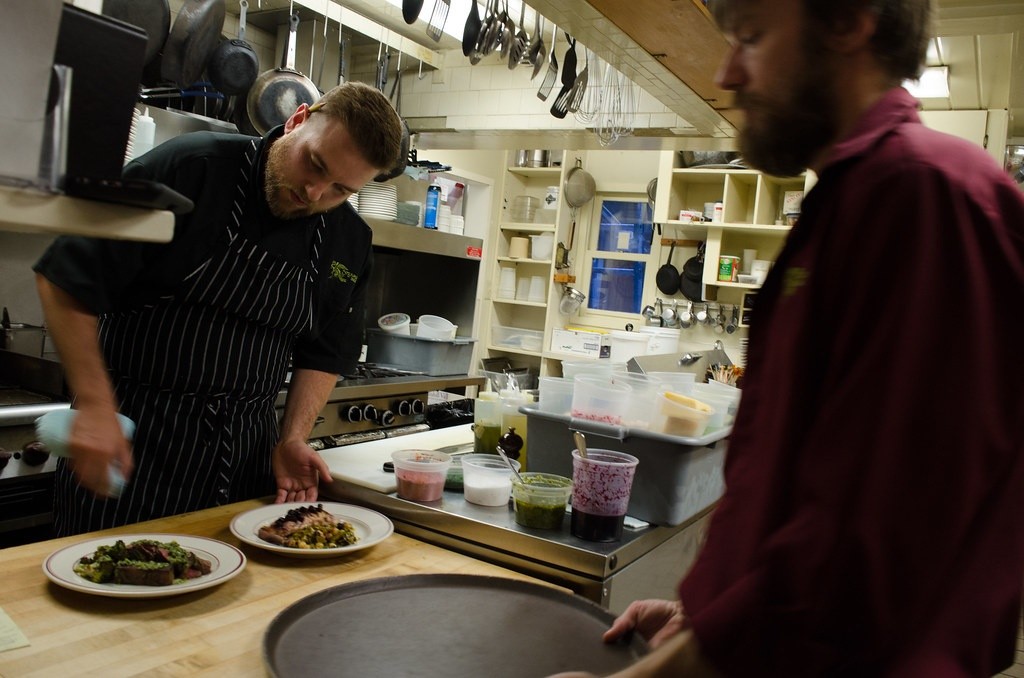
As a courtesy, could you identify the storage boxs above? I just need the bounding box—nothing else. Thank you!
[491,323,543,351]
[515,394,738,530]
[550,326,613,360]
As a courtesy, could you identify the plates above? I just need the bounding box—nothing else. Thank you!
[43,533,247,598]
[123,108,141,165]
[739,338,749,376]
[347,182,397,221]
[230,502,394,554]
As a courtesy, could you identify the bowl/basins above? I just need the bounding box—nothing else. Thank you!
[529,235,554,260]
[391,449,521,506]
[406,201,464,236]
[510,472,573,530]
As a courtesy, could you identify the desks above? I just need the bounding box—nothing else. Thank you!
[0,496,574,678]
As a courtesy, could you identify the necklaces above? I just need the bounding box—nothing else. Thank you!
[551,0,1024,678]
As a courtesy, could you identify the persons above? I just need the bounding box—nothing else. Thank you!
[29,82,405,537]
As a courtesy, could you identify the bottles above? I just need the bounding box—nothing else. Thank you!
[712,201,723,223]
[355,307,368,363]
[133,107,155,161]
[447,183,465,215]
[474,378,533,473]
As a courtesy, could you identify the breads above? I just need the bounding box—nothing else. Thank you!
[259,504,334,544]
[114,562,176,585]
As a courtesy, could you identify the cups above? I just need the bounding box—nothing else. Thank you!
[378,313,410,336]
[538,358,742,437]
[498,267,545,303]
[417,315,454,340]
[572,448,639,543]
[511,196,534,223]
[720,249,771,284]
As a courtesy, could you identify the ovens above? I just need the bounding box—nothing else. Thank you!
[0,351,72,550]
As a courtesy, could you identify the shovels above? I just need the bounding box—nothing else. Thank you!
[537,23,558,101]
[562,37,576,88]
[550,32,576,119]
[426,0,451,42]
[566,44,588,113]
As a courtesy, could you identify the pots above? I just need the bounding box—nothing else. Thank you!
[656,241,706,303]
[374,69,409,182]
[103,0,321,137]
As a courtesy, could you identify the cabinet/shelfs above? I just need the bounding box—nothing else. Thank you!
[485,144,586,395]
[649,151,819,302]
